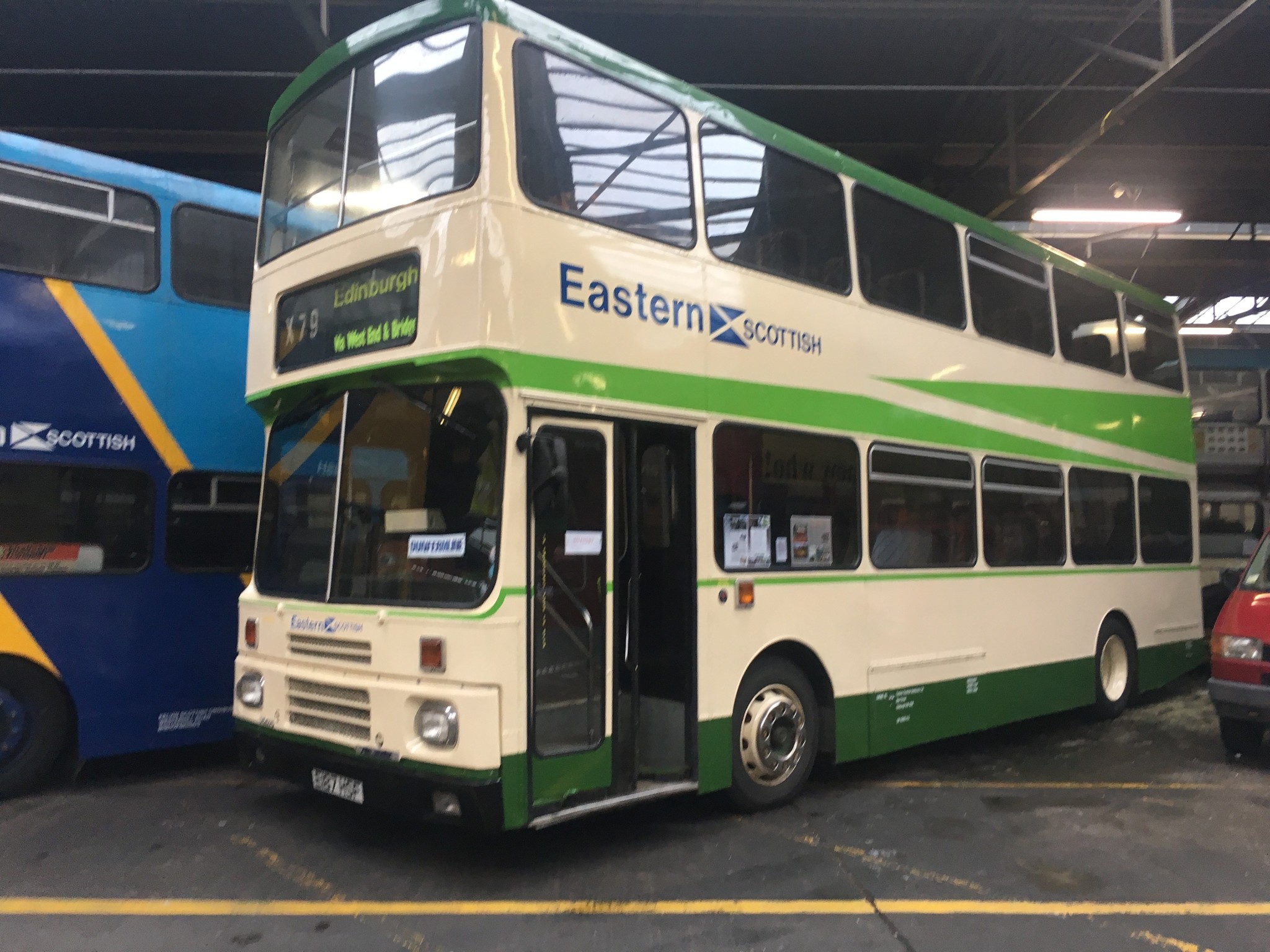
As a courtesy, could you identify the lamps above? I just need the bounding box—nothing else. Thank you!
[993,221,1270,243]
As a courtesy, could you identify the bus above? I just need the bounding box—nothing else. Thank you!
[234,0,1207,837]
[1,129,360,805]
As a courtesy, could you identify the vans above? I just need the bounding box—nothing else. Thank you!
[1204,524,1270,753]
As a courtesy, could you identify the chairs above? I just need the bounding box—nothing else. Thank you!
[735,230,1151,383]
[870,526,941,568]
